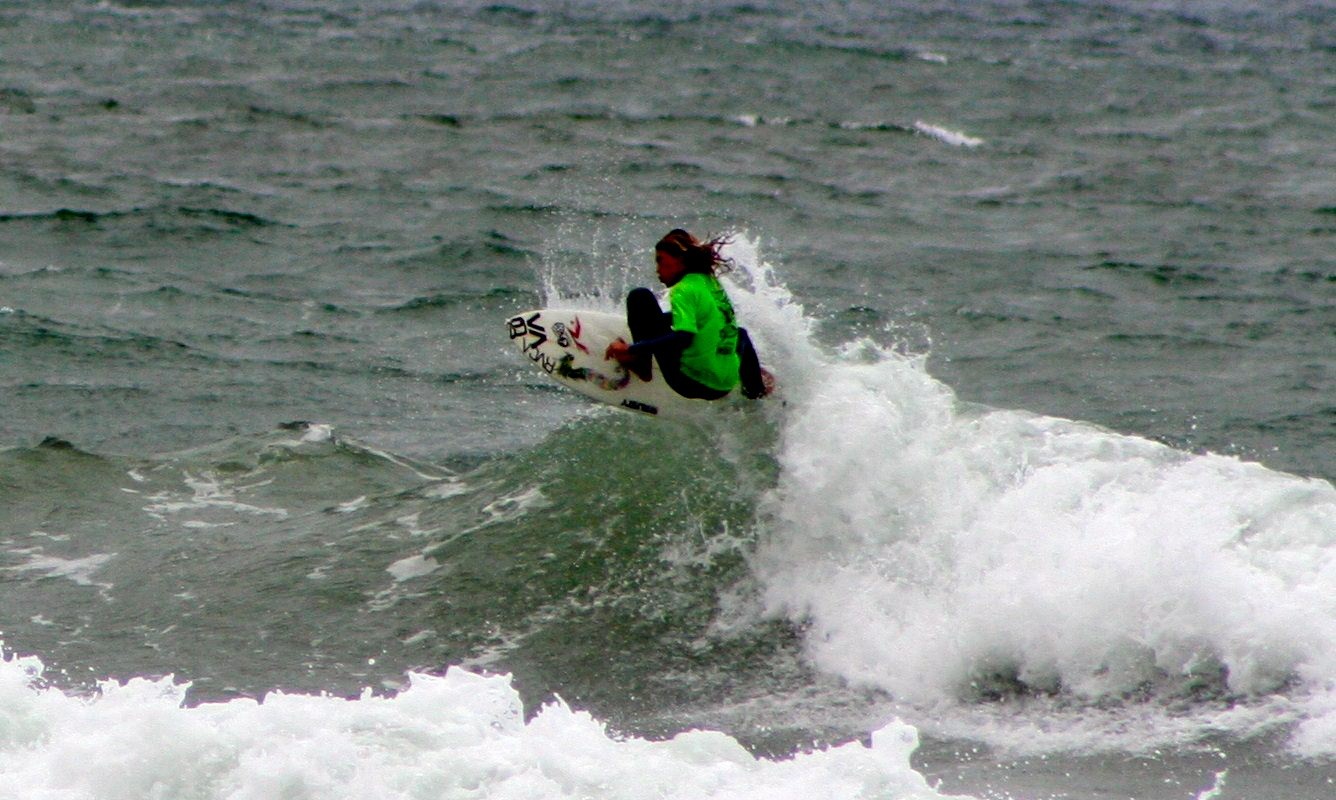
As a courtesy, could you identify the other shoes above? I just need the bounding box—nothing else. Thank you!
[758,365,776,396]
[609,341,653,382]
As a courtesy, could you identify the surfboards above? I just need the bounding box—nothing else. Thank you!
[505,309,774,422]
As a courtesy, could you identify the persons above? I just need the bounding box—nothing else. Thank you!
[606,229,767,400]
